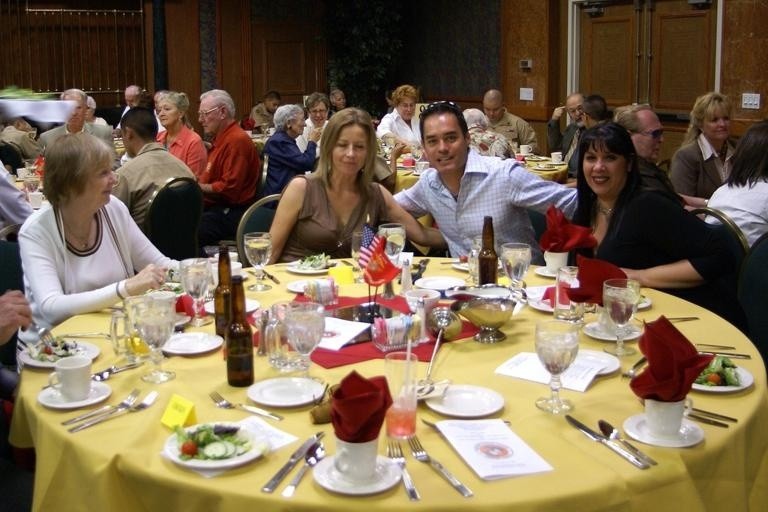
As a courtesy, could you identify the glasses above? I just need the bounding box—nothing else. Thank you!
[629,127,664,139]
[196,104,223,119]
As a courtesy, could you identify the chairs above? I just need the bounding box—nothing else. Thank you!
[9,124,767,365]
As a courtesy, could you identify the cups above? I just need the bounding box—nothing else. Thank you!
[28,191,46,209]
[16,169,26,180]
[385,350,417,440]
[244,131,253,139]
[327,433,379,482]
[414,161,430,174]
[451,298,520,344]
[24,176,41,192]
[269,128,276,136]
[550,151,563,163]
[256,303,286,358]
[46,355,93,402]
[644,398,693,437]
[544,250,569,273]
[554,267,587,325]
[519,144,533,154]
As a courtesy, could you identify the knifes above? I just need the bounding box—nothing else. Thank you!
[563,415,649,470]
[67,391,159,435]
[262,429,325,493]
[165,420,273,470]
[262,268,281,285]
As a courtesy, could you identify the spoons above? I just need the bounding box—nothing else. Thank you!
[597,418,660,466]
[282,440,327,498]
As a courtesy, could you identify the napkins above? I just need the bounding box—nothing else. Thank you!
[532,204,599,254]
[328,369,395,443]
[629,315,716,403]
[570,252,630,306]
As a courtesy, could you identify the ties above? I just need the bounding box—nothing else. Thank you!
[563,127,581,168]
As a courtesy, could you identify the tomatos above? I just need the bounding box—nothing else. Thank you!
[707,374,722,384]
[182,443,196,456]
[460,255,468,263]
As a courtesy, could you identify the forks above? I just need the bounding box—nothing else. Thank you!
[60,387,141,426]
[405,432,474,498]
[387,437,420,499]
[207,390,283,421]
[621,356,649,379]
[632,317,700,326]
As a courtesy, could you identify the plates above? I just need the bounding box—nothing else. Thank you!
[285,258,338,274]
[693,354,756,395]
[623,414,705,447]
[638,293,652,310]
[394,165,414,170]
[19,338,103,369]
[568,349,621,378]
[286,279,337,291]
[412,172,419,176]
[34,381,113,411]
[424,384,505,417]
[516,154,566,171]
[415,273,468,290]
[313,454,404,496]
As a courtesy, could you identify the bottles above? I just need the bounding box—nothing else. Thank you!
[213,240,257,389]
[477,215,498,285]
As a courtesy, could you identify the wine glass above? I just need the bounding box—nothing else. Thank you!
[179,257,213,328]
[602,278,641,355]
[534,319,580,413]
[137,292,176,385]
[246,220,533,293]
[283,302,326,388]
